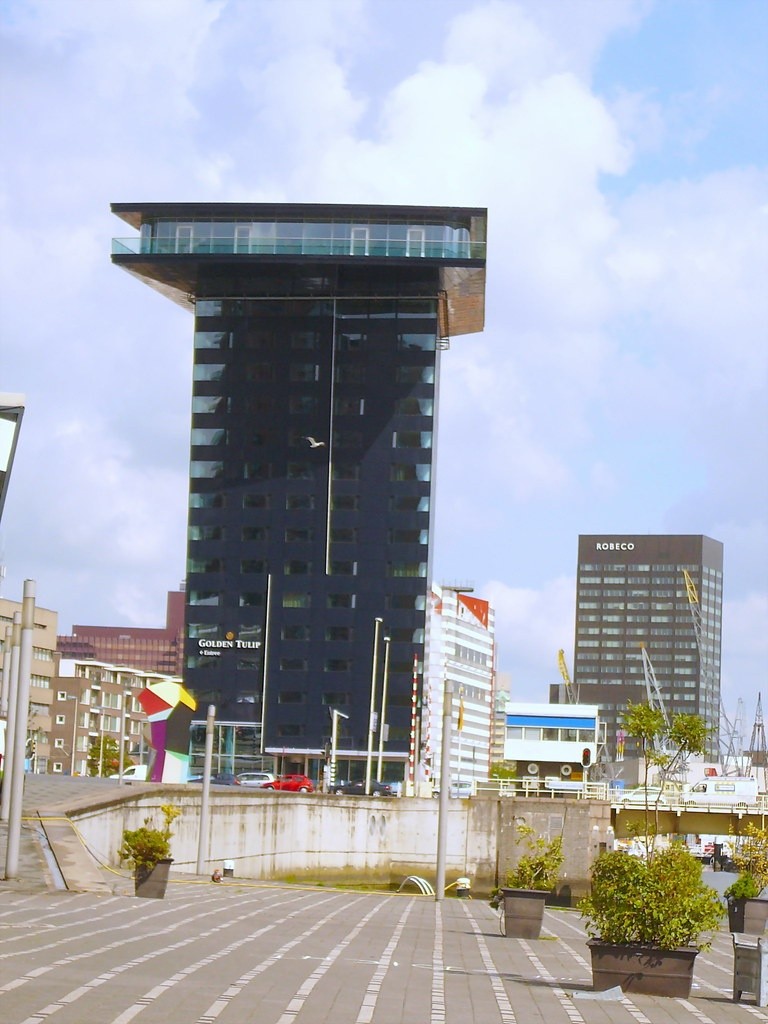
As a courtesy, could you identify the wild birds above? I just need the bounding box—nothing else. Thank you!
[300,436,326,449]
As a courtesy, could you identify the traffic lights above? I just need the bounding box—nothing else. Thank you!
[582,747,592,768]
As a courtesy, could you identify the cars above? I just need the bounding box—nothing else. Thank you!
[432,780,474,800]
[328,777,392,797]
[109,764,149,781]
[384,781,403,796]
[188,773,241,786]
[261,773,314,794]
[618,786,668,807]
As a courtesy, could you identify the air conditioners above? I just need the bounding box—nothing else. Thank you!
[522,776,538,789]
[544,777,559,788]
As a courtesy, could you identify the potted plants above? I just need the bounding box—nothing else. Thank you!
[490,821,566,939]
[576,699,728,999]
[727,820,768,934]
[117,803,183,899]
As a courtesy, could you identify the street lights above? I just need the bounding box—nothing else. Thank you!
[364,616,383,796]
[374,636,391,781]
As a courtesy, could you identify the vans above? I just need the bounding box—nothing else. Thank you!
[679,775,759,810]
[236,772,276,788]
